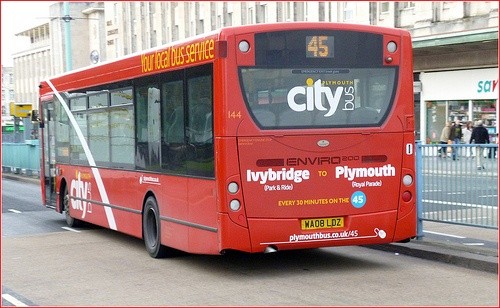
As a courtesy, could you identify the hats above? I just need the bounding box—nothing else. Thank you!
[455,120,460,125]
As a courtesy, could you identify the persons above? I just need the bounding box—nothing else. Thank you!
[440,119,499,170]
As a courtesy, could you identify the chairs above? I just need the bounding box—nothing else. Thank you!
[314,109,347,125]
[278,109,313,126]
[348,107,378,124]
[252,109,275,127]
[203,112,212,143]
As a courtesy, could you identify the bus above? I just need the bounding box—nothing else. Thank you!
[37,21,417,259]
